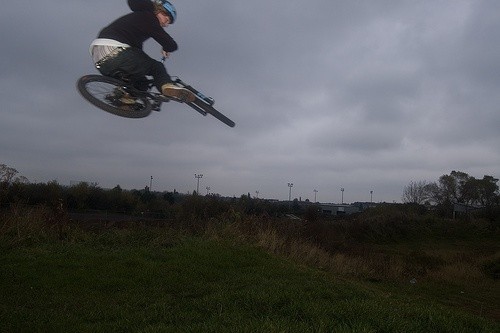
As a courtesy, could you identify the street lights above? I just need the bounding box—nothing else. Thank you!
[288,182,293,201]
[195,174,203,194]
[371,190,373,202]
[340,187,344,203]
[314,189,318,202]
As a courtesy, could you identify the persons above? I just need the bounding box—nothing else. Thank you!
[89,0,196,103]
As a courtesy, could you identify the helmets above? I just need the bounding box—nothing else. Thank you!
[156,0,176,24]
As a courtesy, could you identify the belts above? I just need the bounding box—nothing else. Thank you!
[94,47,125,69]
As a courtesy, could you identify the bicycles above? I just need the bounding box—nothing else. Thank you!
[77,50,237,130]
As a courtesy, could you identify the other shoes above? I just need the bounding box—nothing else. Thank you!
[120,97,143,104]
[162,83,196,103]
